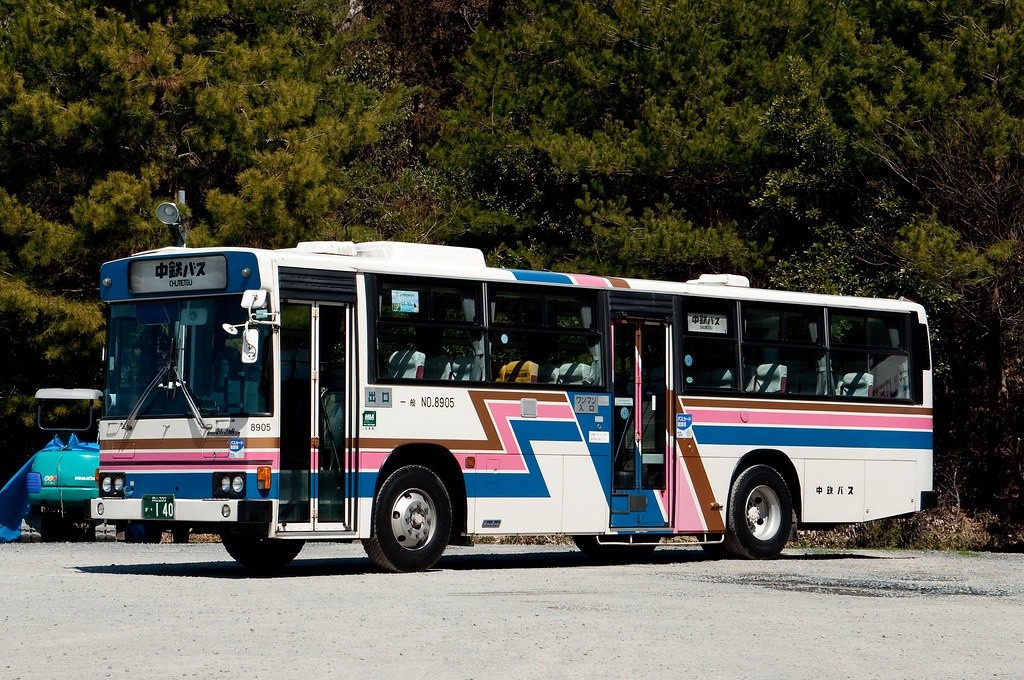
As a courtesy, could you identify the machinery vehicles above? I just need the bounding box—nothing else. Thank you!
[26,388,190,544]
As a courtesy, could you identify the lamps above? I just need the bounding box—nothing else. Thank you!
[156,201,180,226]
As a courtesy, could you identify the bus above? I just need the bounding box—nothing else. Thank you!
[90,239,934,573]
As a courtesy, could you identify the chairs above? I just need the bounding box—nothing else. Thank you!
[490,361,508,383]
[538,363,560,384]
[798,372,824,396]
[383,348,428,380]
[505,359,538,383]
[832,372,844,396]
[843,371,875,398]
[743,365,760,393]
[754,363,788,393]
[450,355,482,381]
[424,355,455,381]
[558,362,594,385]
[710,367,736,388]
